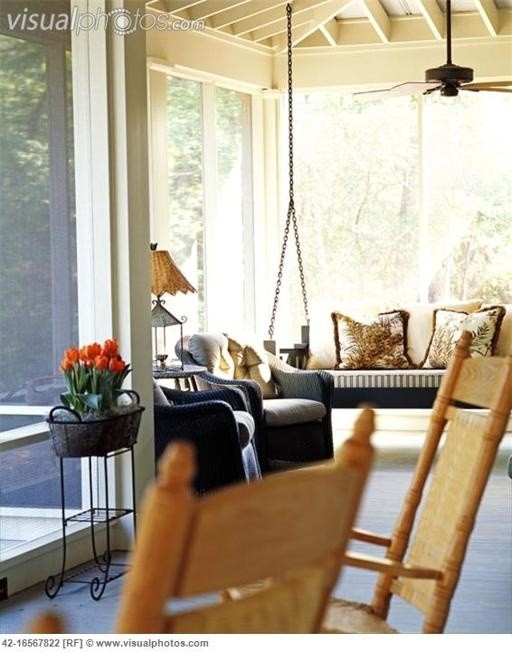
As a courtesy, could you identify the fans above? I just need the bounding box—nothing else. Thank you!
[349,0,512,97]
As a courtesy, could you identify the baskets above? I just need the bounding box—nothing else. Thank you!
[47,390,145,457]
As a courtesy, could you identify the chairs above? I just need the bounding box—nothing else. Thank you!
[152,377,263,501]
[174,329,333,479]
[26,408,378,633]
[315,333,512,633]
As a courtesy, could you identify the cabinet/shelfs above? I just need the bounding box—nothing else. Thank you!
[44,448,137,600]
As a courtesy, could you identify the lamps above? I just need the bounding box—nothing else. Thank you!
[150,243,198,371]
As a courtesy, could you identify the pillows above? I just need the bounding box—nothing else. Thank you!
[307,301,506,370]
[188,331,280,398]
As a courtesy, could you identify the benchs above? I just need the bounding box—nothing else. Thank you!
[265,326,512,408]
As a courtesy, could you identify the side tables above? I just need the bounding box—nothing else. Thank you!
[152,361,208,393]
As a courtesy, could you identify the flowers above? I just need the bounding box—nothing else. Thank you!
[59,341,133,414]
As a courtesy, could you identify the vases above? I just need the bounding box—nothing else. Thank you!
[49,405,144,459]
